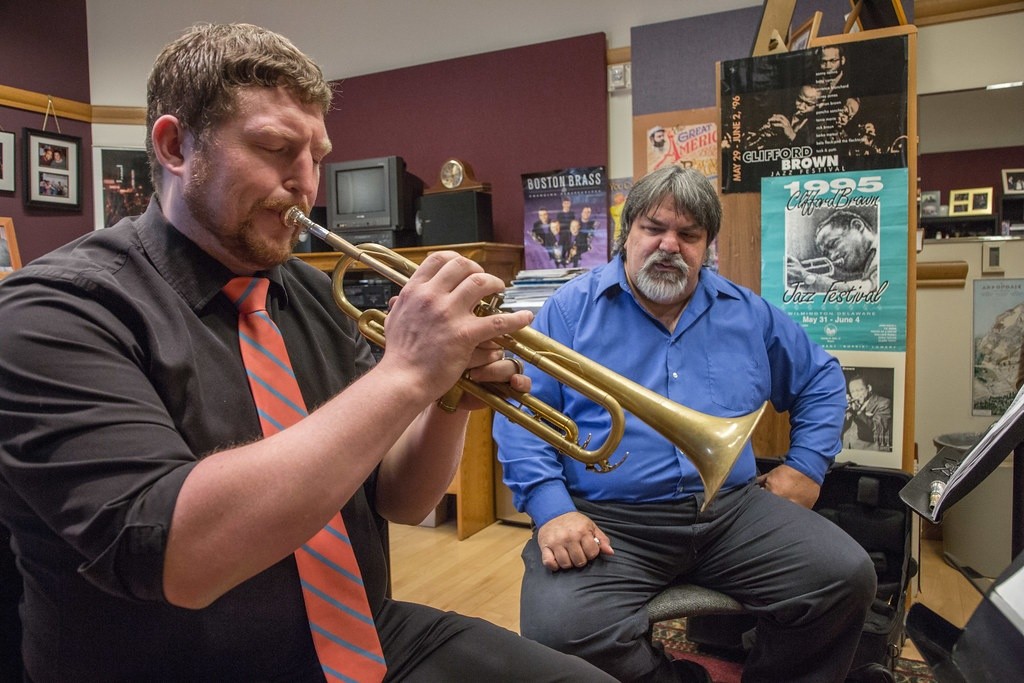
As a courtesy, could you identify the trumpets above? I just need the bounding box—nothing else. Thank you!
[843,398,859,411]
[787,255,846,288]
[282,203,769,514]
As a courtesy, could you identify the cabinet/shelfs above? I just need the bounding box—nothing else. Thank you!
[918,212,999,240]
[285,241,524,542]
[997,193,1024,237]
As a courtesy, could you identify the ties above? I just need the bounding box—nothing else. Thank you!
[221,277,387,683]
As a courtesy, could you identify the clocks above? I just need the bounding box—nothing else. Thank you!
[440,160,463,190]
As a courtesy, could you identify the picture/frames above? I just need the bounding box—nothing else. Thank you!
[842,0,908,33]
[785,11,823,53]
[1001,167,1024,195]
[0,217,23,282]
[948,187,994,216]
[0,130,17,197]
[22,126,84,213]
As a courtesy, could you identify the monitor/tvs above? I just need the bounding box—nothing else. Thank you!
[324,155,425,233]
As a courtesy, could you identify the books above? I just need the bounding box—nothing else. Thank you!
[497,269,582,318]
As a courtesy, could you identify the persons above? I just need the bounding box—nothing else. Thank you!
[0,19,621,683]
[38,173,69,198]
[816,44,849,108]
[785,209,878,296]
[38,142,69,171]
[841,372,892,451]
[831,93,876,148]
[758,83,824,148]
[491,164,878,683]
[532,198,596,268]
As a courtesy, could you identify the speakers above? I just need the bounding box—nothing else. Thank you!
[420,190,494,247]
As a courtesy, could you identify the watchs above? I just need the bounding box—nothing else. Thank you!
[803,273,820,288]
